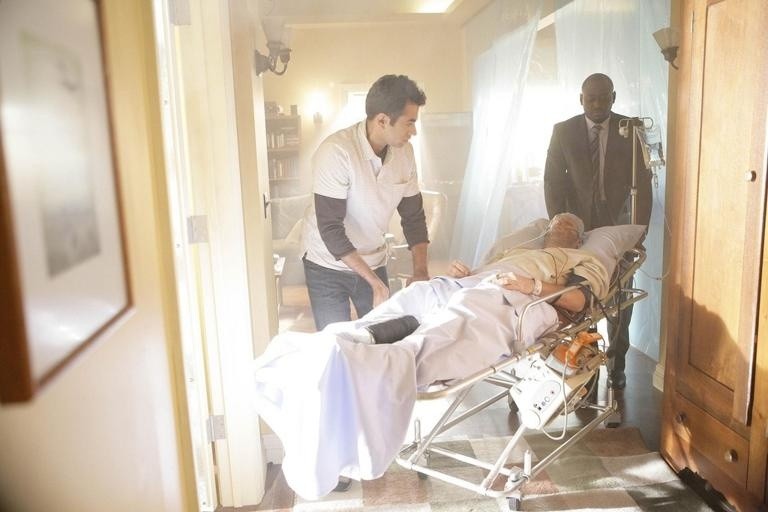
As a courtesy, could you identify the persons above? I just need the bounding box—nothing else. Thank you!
[540,72,655,430]
[443,205,612,377]
[297,74,433,331]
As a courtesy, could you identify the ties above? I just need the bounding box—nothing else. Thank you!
[588,125,604,209]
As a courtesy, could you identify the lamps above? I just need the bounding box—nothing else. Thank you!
[254,41,292,77]
[653,28,682,73]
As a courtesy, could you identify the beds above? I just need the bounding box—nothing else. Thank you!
[241,219,648,512]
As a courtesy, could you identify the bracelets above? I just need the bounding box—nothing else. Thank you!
[534,278,544,296]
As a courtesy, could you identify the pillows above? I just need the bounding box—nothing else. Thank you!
[490,218,649,278]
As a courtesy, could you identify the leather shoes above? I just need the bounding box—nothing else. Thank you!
[606,371,625,390]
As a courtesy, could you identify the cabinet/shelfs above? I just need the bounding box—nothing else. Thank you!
[657,0,768,511]
[266,114,314,181]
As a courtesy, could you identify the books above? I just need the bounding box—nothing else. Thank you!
[264,103,305,199]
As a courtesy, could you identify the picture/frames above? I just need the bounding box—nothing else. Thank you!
[1,0,134,405]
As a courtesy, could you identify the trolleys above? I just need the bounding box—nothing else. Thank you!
[390,246,651,512]
